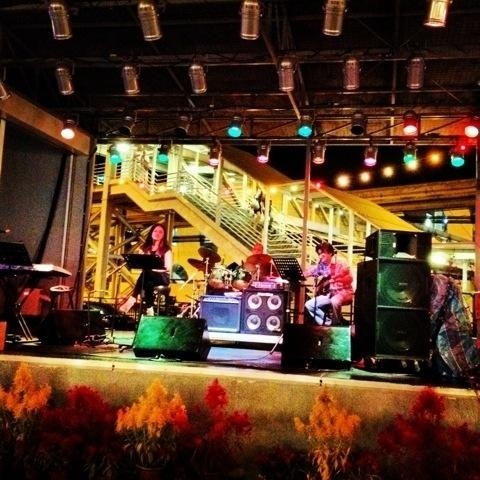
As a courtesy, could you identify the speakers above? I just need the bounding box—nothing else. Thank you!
[280,324,352,373]
[133,313,211,363]
[241,288,292,337]
[357,231,431,359]
[199,295,241,334]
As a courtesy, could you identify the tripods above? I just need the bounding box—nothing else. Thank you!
[269,280,307,355]
[119,270,167,353]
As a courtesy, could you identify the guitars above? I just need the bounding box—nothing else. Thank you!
[311,266,352,291]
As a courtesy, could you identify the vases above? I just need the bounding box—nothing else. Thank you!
[138,465,165,479]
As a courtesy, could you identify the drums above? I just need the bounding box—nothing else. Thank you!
[231,270,252,292]
[206,268,234,292]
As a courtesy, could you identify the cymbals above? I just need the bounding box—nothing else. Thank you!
[187,257,212,275]
[246,254,275,273]
[197,246,222,265]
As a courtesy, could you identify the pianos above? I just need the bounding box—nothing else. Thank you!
[0,263,71,278]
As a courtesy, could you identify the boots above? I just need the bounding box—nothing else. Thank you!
[146,307,154,315]
[119,295,136,317]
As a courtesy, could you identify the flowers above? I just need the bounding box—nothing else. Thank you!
[115,382,184,467]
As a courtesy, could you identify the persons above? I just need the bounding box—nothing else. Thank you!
[253,243,263,254]
[304,242,354,326]
[119,222,173,316]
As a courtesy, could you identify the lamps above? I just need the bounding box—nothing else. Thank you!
[365,146,378,166]
[406,158,420,172]
[350,109,366,137]
[118,107,139,140]
[462,121,479,140]
[208,141,222,167]
[406,53,425,91]
[358,170,371,184]
[227,112,244,139]
[342,55,360,92]
[157,140,172,162]
[335,172,352,190]
[56,67,73,96]
[60,111,79,140]
[239,0,260,40]
[136,2,163,43]
[48,3,72,40]
[425,1,451,28]
[383,166,394,179]
[404,142,418,165]
[278,56,295,93]
[429,150,442,167]
[323,1,345,36]
[298,111,318,137]
[187,62,207,94]
[122,65,139,96]
[403,109,421,137]
[174,111,191,139]
[257,140,272,165]
[311,140,328,164]
[451,148,465,168]
[107,142,120,162]
